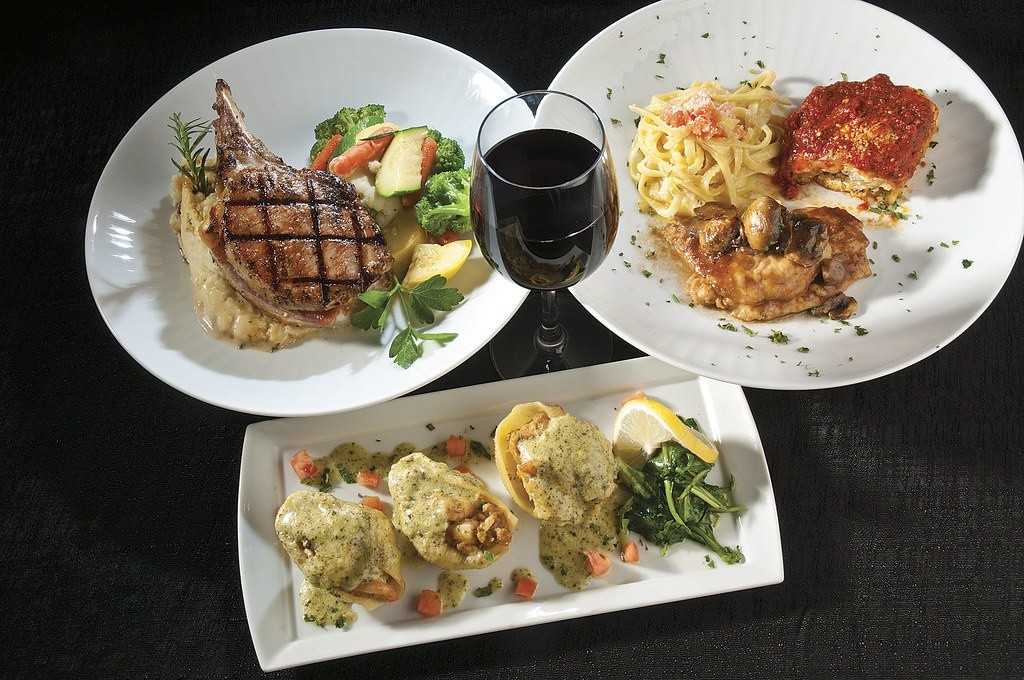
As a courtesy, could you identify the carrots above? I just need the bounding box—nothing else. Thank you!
[311,121,461,245]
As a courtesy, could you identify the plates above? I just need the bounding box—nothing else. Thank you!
[233,354,784,672]
[84,34,535,419]
[536,2,1024,390]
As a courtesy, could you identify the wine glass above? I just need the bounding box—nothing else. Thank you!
[472,90,623,373]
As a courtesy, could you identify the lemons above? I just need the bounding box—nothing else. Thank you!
[612,401,718,470]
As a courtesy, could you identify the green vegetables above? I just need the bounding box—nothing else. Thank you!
[622,440,747,567]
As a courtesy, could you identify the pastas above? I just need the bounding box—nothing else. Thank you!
[631,69,780,219]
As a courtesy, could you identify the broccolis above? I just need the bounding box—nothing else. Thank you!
[309,98,471,238]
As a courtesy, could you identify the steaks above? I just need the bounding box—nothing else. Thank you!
[208,71,394,329]
